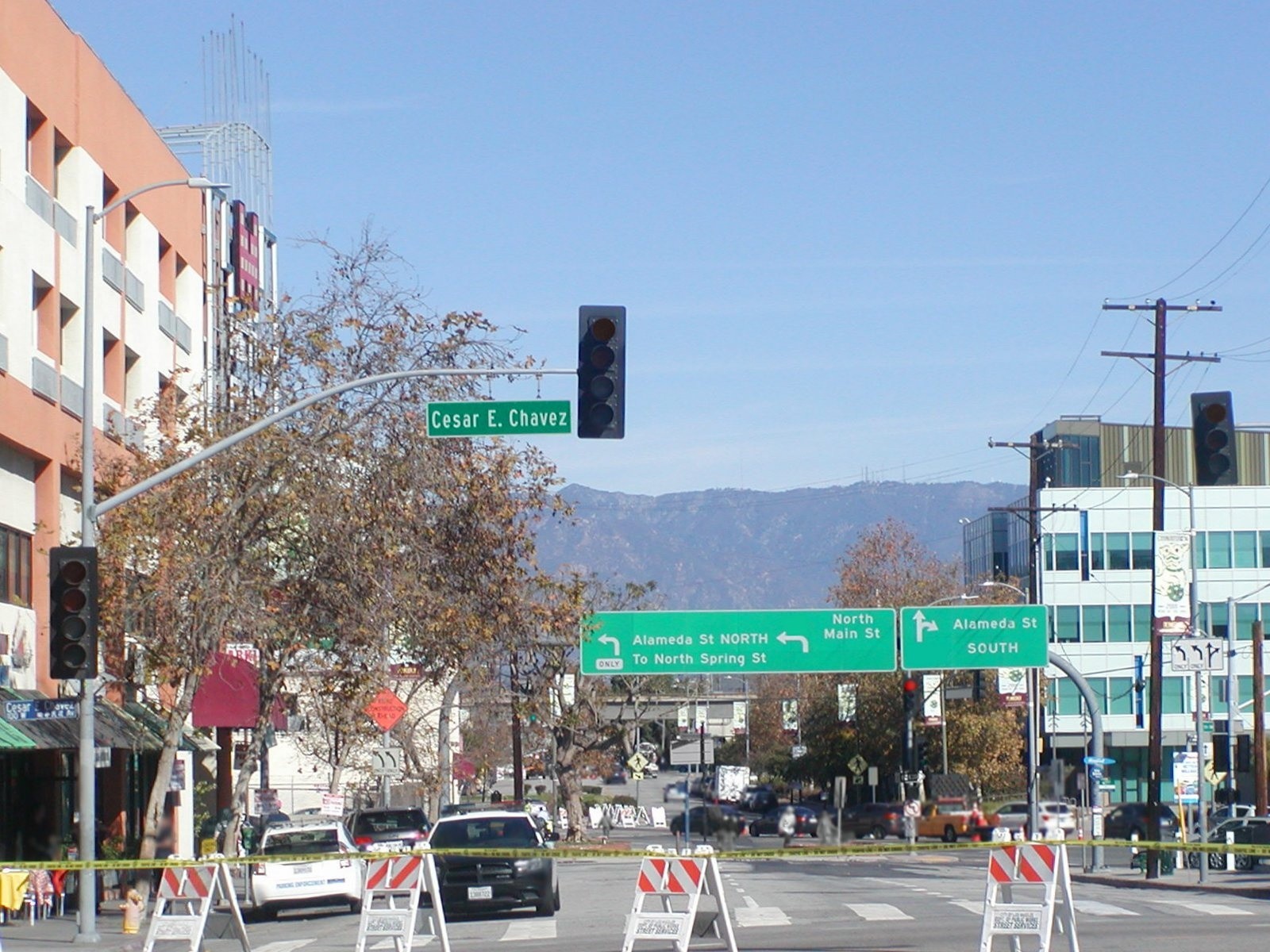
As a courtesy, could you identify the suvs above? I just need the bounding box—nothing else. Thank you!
[1104,802,1181,842]
[338,803,434,859]
[993,800,1077,841]
[843,803,905,840]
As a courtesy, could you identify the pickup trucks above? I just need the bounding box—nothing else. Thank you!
[916,795,997,843]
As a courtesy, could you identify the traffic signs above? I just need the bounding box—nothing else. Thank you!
[900,605,1050,670]
[3,696,80,723]
[894,769,925,783]
[577,608,896,676]
[427,400,571,435]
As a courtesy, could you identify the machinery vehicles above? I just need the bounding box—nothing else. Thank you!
[629,740,659,780]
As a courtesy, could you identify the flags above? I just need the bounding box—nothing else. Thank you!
[1149,528,1194,638]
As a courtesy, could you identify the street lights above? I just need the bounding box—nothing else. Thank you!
[979,579,1039,838]
[73,176,232,943]
[1114,469,1213,883]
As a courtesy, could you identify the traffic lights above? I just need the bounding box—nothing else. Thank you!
[1193,390,1238,485]
[48,545,100,681]
[577,304,627,440]
[901,678,923,718]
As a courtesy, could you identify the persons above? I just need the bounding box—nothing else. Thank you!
[598,810,612,840]
[538,806,549,838]
[524,800,532,812]
[815,810,838,845]
[776,805,797,847]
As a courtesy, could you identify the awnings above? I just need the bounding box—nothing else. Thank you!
[0,686,222,760]
[191,650,290,732]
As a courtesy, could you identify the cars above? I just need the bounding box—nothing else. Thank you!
[691,774,714,801]
[748,803,819,838]
[602,764,627,784]
[669,805,746,837]
[248,819,368,923]
[1186,803,1270,869]
[663,783,686,803]
[427,798,562,923]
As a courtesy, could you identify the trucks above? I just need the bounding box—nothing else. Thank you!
[715,765,750,804]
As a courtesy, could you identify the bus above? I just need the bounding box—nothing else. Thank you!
[523,749,550,779]
